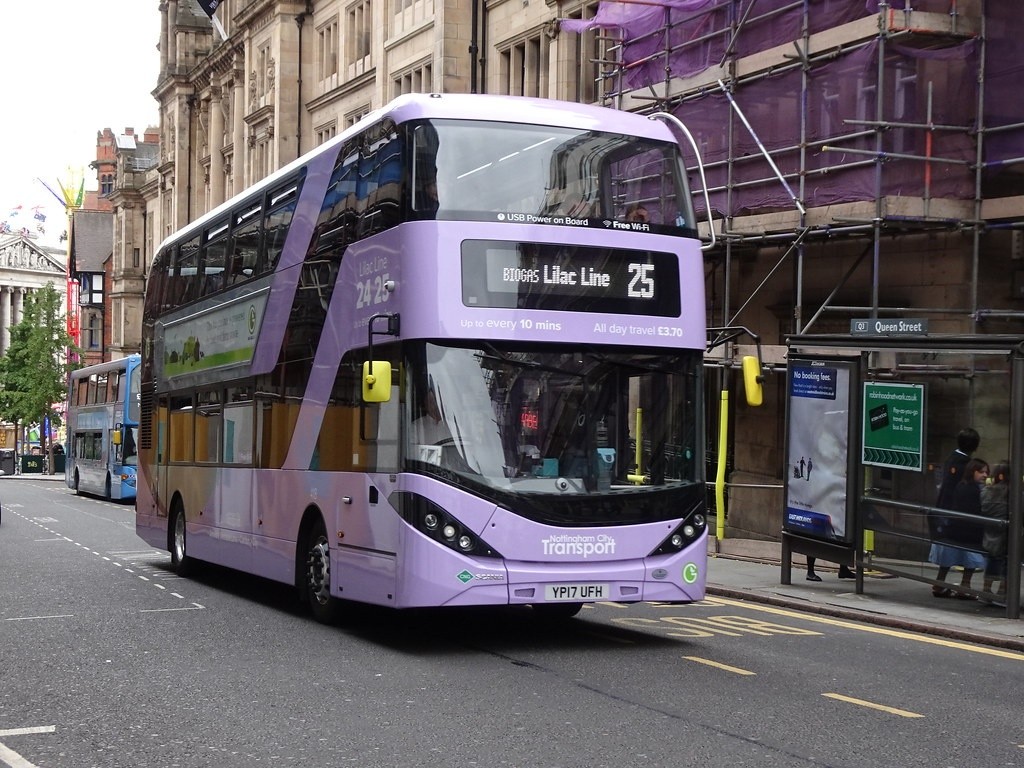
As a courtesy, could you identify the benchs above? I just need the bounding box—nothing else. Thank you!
[864,520,1008,572]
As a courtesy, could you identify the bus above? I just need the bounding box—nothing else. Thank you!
[136,92,768,619]
[65,354,140,501]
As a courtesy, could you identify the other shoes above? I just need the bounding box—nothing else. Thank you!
[992,594,1007,607]
[932,587,956,597]
[955,591,978,599]
[806,572,822,581]
[838,570,857,578]
[978,590,994,604]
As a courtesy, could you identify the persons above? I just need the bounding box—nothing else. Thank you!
[928,427,1009,607]
[407,387,470,459]
[806,555,857,581]
[794,456,813,481]
